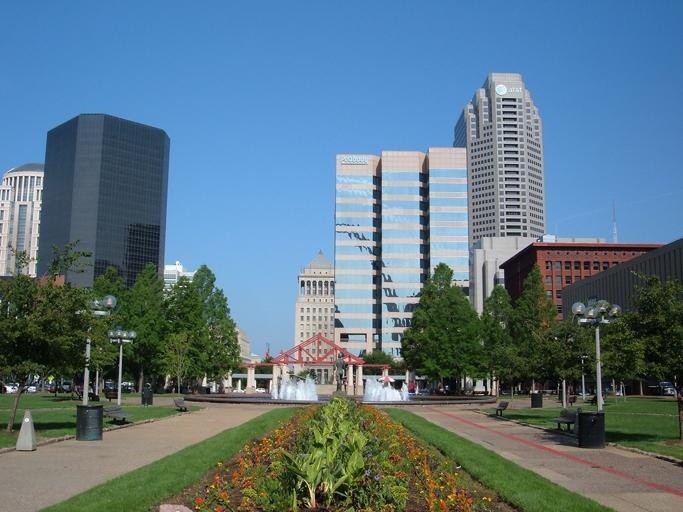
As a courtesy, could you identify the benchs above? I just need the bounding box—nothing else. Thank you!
[491,400,509,418]
[553,391,606,407]
[101,406,133,422]
[71,384,118,403]
[550,405,583,432]
[173,398,190,412]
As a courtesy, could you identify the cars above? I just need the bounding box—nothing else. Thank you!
[517,384,626,396]
[1,376,265,400]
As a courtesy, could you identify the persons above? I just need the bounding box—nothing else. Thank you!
[333,352,345,391]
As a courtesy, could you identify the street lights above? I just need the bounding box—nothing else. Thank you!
[576,355,588,401]
[106,327,135,405]
[80,291,116,407]
[572,297,621,415]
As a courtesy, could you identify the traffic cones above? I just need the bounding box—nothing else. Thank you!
[14,408,38,452]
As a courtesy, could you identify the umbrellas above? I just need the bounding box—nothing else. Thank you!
[377,376,395,382]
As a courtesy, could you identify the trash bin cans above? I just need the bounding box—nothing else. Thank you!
[576,407,605,448]
[531,392,542,408]
[142,383,153,408]
[76,404,103,441]
[559,391,562,400]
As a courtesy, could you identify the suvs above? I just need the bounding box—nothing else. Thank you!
[656,381,673,396]
